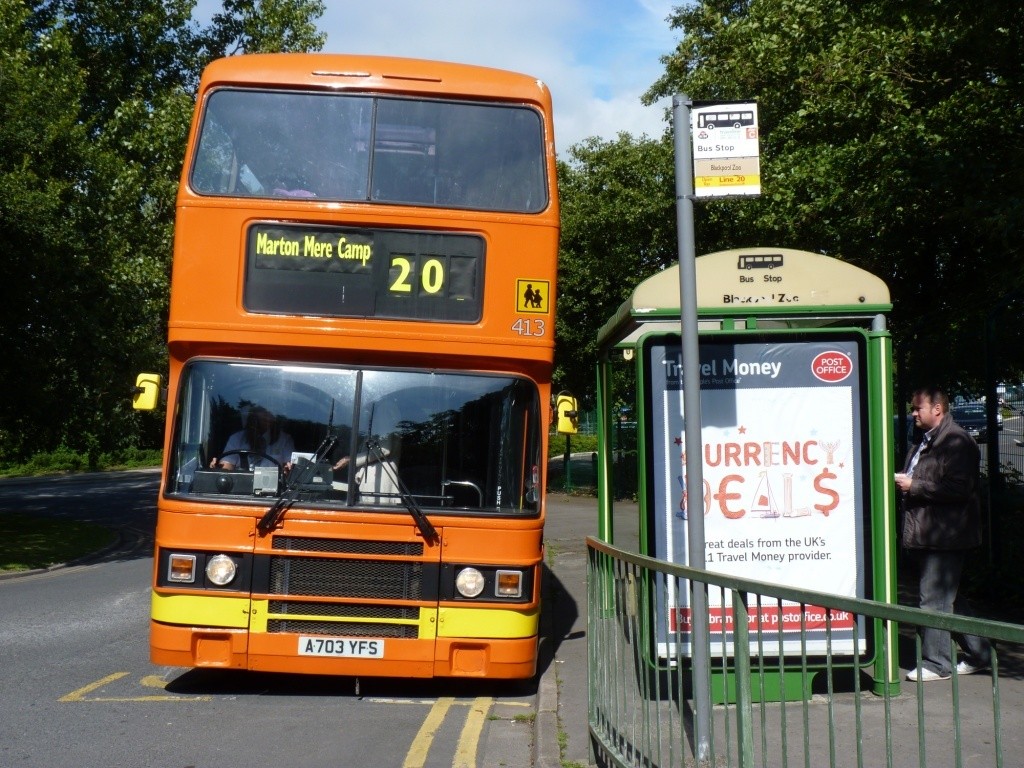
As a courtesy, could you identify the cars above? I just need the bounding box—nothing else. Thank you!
[948,384,1024,442]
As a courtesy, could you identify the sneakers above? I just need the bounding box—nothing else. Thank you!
[957,660,992,675]
[905,666,951,682]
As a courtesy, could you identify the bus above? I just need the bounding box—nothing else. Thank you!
[127,54,579,678]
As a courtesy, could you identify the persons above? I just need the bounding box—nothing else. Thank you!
[894,382,1000,683]
[336,397,402,504]
[209,403,294,474]
